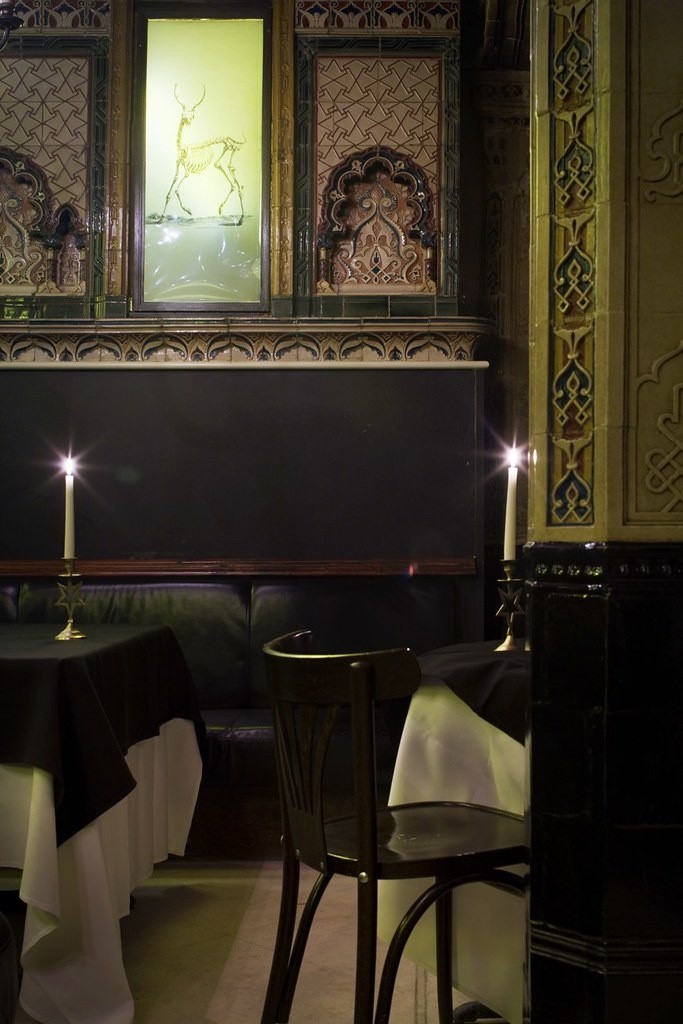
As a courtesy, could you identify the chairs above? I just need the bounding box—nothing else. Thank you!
[260,629,530,1024]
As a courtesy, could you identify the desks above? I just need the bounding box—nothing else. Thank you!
[0,621,172,1024]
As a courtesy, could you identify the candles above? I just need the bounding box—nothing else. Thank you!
[63,458,76,559]
[503,448,519,560]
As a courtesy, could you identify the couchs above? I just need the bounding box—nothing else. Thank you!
[0,572,484,860]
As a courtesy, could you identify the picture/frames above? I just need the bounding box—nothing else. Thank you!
[0,34,110,305]
[124,0,272,313]
[293,27,462,318]
